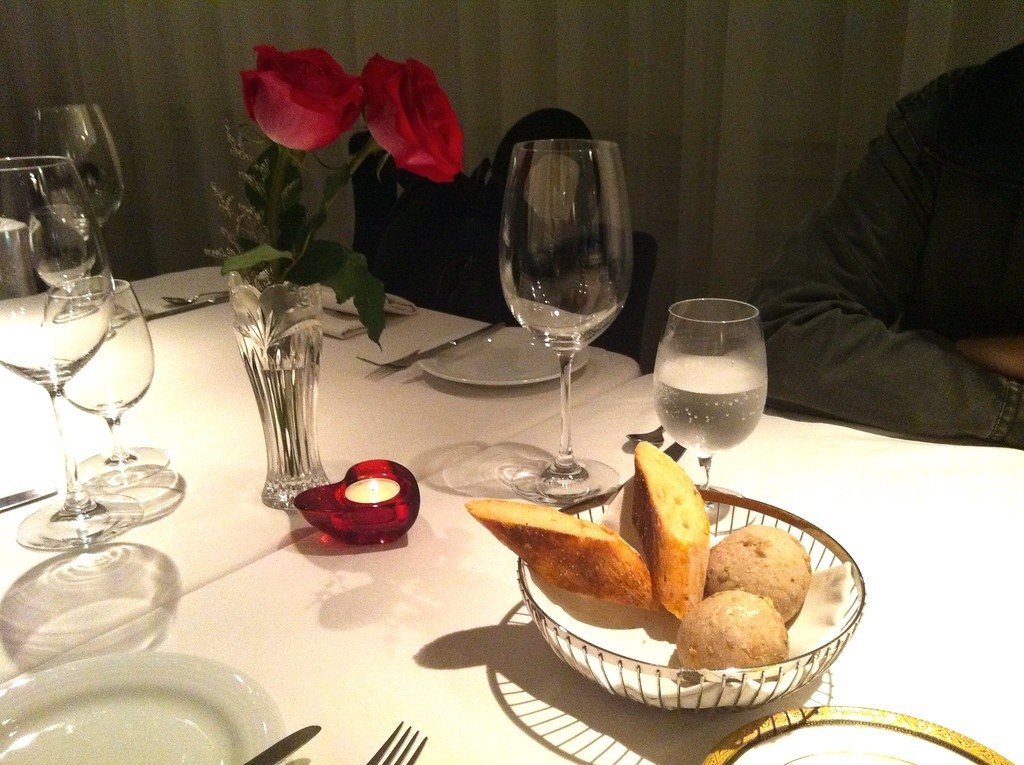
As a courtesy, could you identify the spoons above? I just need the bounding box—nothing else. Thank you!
[162,290,231,306]
[625,425,667,447]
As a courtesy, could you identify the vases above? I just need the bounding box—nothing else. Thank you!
[223,273,331,510]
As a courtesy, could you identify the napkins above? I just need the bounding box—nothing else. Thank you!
[319,283,418,318]
[316,310,367,340]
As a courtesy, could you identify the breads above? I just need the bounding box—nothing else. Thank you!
[465,498,666,612]
[677,591,790,670]
[631,439,710,622]
[702,523,812,622]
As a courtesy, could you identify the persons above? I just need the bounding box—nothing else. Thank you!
[733,37,1024,450]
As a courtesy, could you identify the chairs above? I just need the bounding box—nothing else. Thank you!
[373,108,658,364]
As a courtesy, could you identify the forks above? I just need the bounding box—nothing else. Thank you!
[356,320,507,371]
[365,721,429,765]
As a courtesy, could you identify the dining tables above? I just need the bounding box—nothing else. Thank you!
[2,267,1024,765]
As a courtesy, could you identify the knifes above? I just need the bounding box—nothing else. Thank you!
[0,488,58,511]
[244,725,321,765]
[320,305,359,322]
[140,295,232,321]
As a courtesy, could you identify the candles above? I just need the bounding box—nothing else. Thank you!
[346,477,400,504]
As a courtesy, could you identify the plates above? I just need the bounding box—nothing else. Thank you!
[700,706,1016,765]
[418,327,586,385]
[0,653,289,765]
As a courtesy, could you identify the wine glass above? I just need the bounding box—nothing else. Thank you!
[0,102,171,550]
[653,298,765,536]
[497,138,633,505]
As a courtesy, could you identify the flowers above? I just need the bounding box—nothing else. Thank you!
[204,41,464,353]
